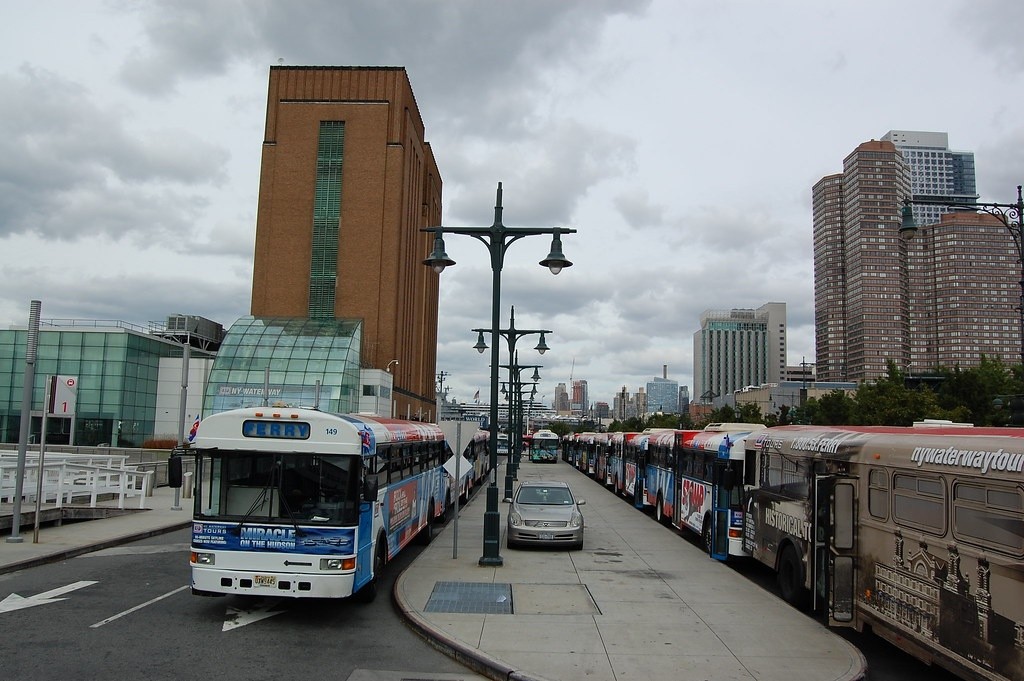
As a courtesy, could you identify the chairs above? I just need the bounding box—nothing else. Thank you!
[550,490,562,503]
[523,489,536,502]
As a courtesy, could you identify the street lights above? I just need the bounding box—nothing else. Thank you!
[418,183,579,566]
[470,304,554,507]
[898,185,1024,375]
[498,380,540,480]
[488,349,544,481]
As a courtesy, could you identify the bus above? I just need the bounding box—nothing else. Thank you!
[560,423,769,565]
[496,433,509,455]
[167,406,490,604]
[741,421,1024,681]
[528,429,563,463]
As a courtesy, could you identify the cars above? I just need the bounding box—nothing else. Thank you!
[502,481,586,550]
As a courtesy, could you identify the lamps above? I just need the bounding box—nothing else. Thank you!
[386,359,399,372]
